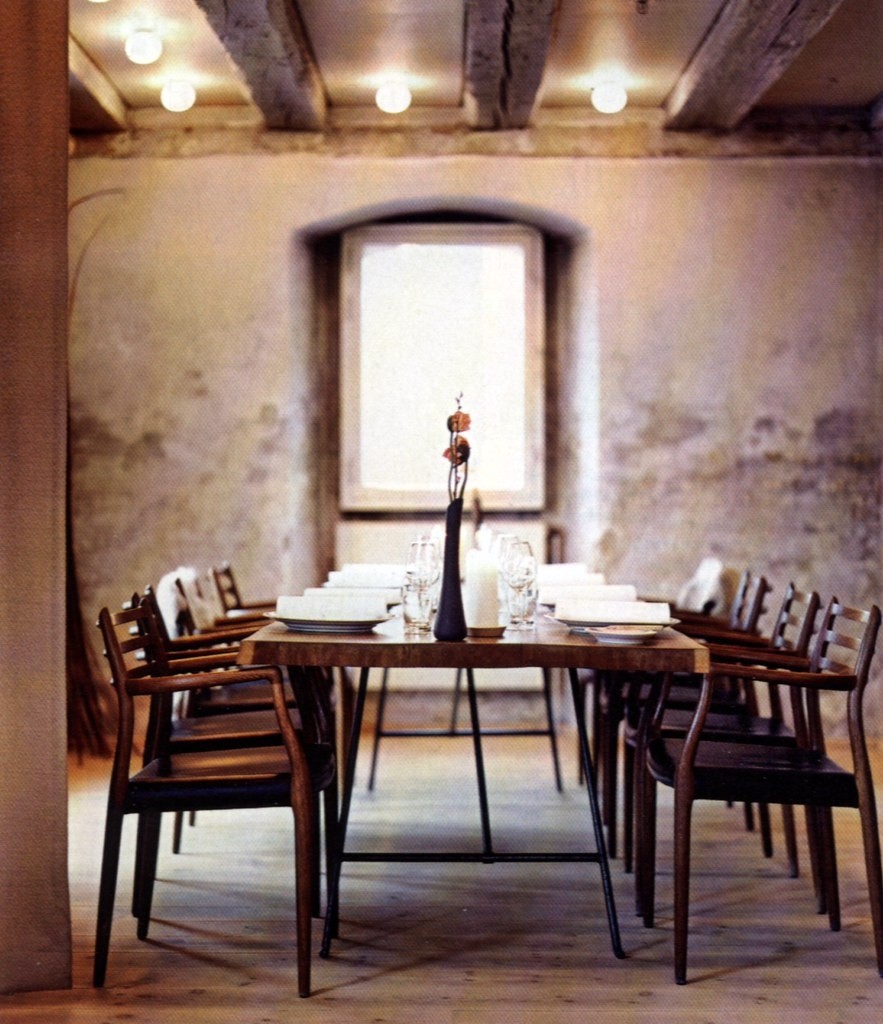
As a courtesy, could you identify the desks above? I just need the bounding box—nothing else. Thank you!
[237,590,710,999]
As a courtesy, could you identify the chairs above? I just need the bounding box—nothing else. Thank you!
[91,562,335,999]
[562,560,883,989]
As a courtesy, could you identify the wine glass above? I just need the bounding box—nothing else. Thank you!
[400,535,441,635]
[497,533,539,629]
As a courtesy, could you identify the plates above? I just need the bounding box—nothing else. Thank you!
[264,560,403,634]
[535,560,681,646]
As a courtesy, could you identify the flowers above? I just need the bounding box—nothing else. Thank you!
[443,391,471,504]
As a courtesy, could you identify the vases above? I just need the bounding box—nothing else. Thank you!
[432,496,469,641]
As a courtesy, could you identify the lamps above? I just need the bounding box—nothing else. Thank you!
[374,83,411,114]
[591,82,628,114]
[161,78,197,112]
[122,30,162,63]
